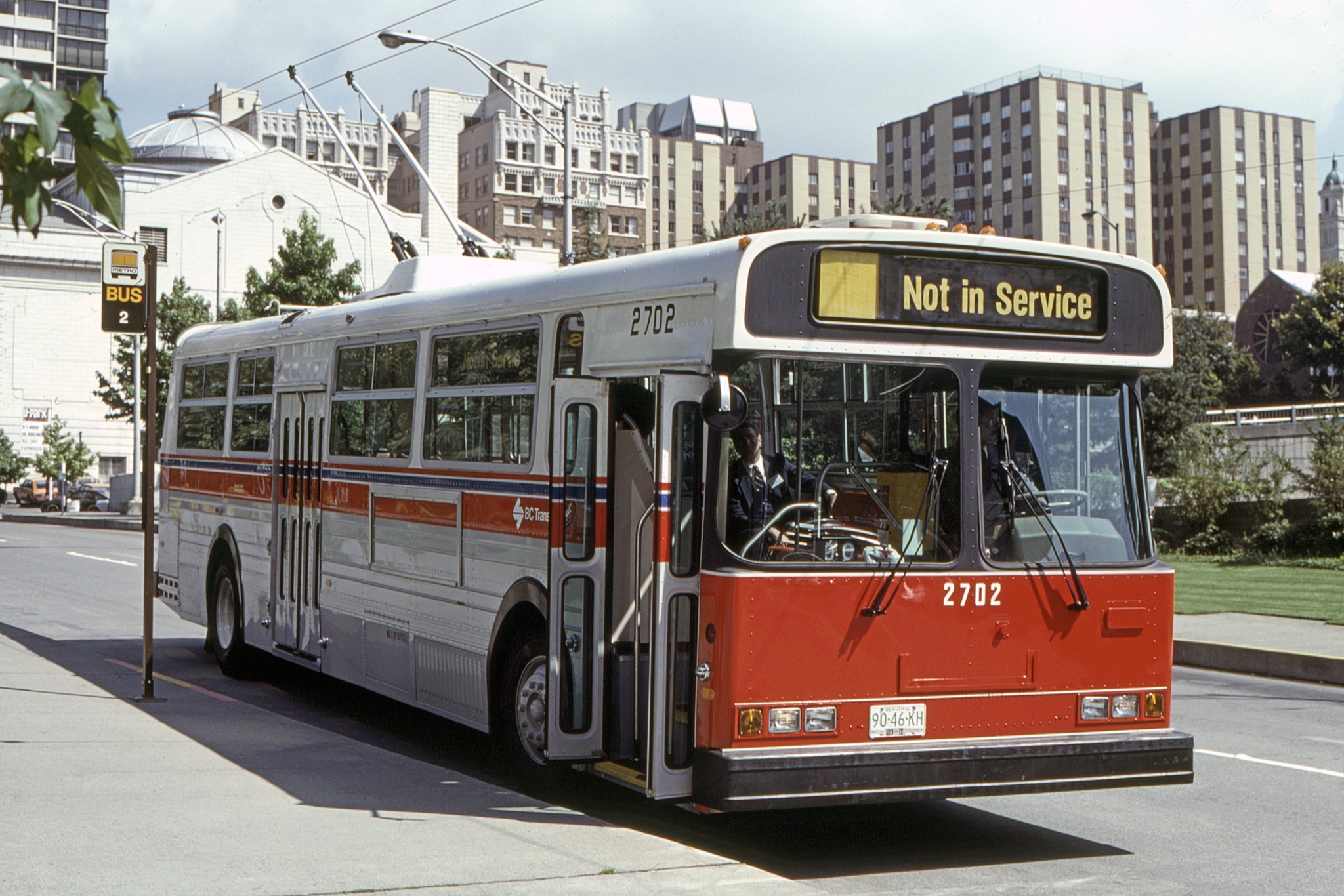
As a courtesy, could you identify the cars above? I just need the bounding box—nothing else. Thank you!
[71,478,111,491]
[41,489,110,513]
[12,476,75,507]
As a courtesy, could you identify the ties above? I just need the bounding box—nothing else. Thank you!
[751,466,764,492]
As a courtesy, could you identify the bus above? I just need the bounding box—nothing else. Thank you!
[152,63,1196,817]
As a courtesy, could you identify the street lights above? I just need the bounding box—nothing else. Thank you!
[211,214,224,321]
[1081,209,1121,254]
[378,29,574,266]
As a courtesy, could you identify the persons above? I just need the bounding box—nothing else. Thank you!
[920,385,1029,553]
[849,426,883,473]
[728,423,838,558]
